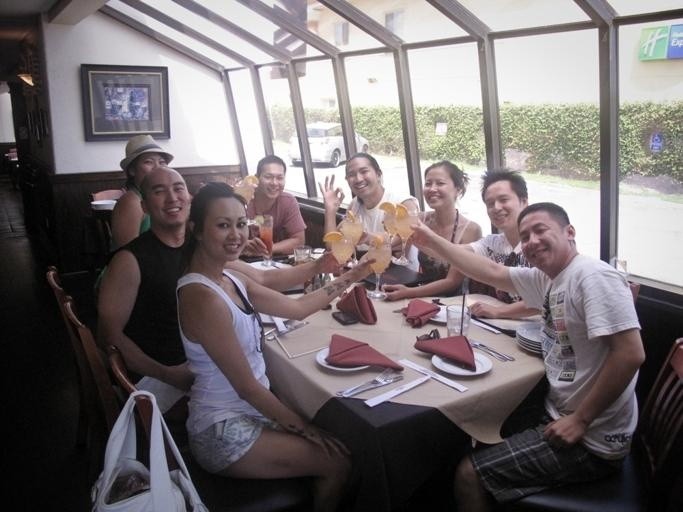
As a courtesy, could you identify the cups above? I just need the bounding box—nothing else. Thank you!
[292,244,312,266]
[257,215,273,265]
[445,303,469,338]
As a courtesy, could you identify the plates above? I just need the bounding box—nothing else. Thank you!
[515,322,541,356]
[258,312,288,324]
[429,305,448,323]
[314,347,368,373]
[89,199,115,210]
[431,350,492,377]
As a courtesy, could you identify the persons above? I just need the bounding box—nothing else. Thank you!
[172,181,379,511]
[408,199,647,509]
[98,132,529,446]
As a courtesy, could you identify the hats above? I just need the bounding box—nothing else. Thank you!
[121,134,174,168]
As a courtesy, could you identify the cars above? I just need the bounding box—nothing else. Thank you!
[289,120,369,168]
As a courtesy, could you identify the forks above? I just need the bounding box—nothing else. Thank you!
[335,367,392,395]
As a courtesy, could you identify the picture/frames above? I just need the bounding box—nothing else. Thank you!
[80,62,170,142]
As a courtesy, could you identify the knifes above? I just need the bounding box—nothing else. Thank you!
[342,376,404,398]
[267,321,309,343]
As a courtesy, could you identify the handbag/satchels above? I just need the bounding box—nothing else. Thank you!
[90,460,211,512]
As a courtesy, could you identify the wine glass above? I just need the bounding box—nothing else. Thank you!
[327,207,420,302]
